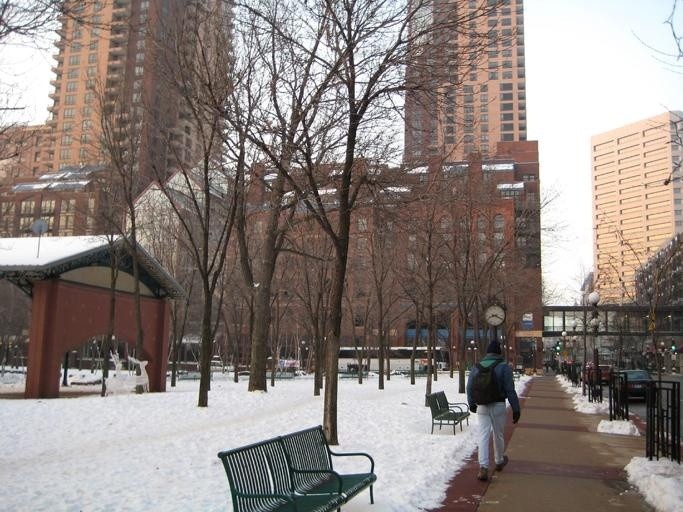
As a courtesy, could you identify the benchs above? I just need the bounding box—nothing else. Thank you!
[217,425,378,512]
[425,391,471,435]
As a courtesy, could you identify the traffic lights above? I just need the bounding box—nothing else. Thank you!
[671,339,675,351]
[555,341,560,351]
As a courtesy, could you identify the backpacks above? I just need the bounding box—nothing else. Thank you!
[473,360,503,404]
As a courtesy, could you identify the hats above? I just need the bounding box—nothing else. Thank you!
[486,341,501,354]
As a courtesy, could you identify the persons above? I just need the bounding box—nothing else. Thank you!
[465,338,521,481]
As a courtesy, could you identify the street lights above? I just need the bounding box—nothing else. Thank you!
[466,340,476,367]
[299,340,308,370]
[561,331,567,375]
[587,291,599,388]
[589,318,598,384]
[570,319,577,386]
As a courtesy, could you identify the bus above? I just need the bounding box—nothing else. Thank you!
[337,346,448,375]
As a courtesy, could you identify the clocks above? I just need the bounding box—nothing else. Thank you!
[483,295,507,328]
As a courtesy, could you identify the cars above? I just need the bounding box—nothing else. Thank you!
[612,369,653,404]
[587,365,610,385]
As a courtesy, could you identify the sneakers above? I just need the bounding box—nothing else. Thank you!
[496,456,508,470]
[477,467,487,480]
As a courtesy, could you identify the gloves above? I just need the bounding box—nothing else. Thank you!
[470,404,477,413]
[513,412,520,424]
